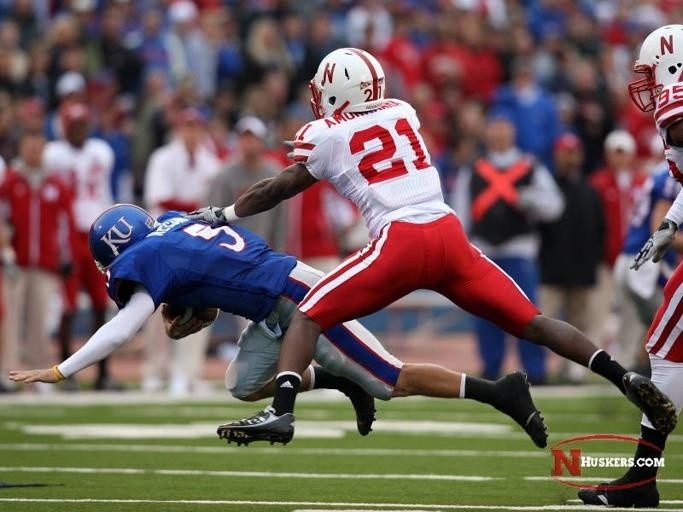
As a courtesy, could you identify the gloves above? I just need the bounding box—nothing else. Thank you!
[628,216,676,272]
[184,204,229,230]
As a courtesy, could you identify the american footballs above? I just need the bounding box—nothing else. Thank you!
[161,303,220,328]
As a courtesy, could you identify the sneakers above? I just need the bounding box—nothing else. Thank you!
[577,475,660,509]
[497,370,548,451]
[343,380,377,436]
[215,408,296,447]
[622,369,678,435]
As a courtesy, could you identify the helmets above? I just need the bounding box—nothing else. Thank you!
[626,24,683,114]
[87,203,160,273]
[307,47,386,119]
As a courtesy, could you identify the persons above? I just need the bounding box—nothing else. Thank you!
[577,21,682,509]
[6,202,549,450]
[0,0,682,386]
[180,45,682,449]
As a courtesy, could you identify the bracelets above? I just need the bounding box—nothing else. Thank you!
[663,186,682,226]
[51,365,65,381]
[221,202,242,224]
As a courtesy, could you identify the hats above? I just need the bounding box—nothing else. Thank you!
[53,0,270,143]
[475,104,641,159]
